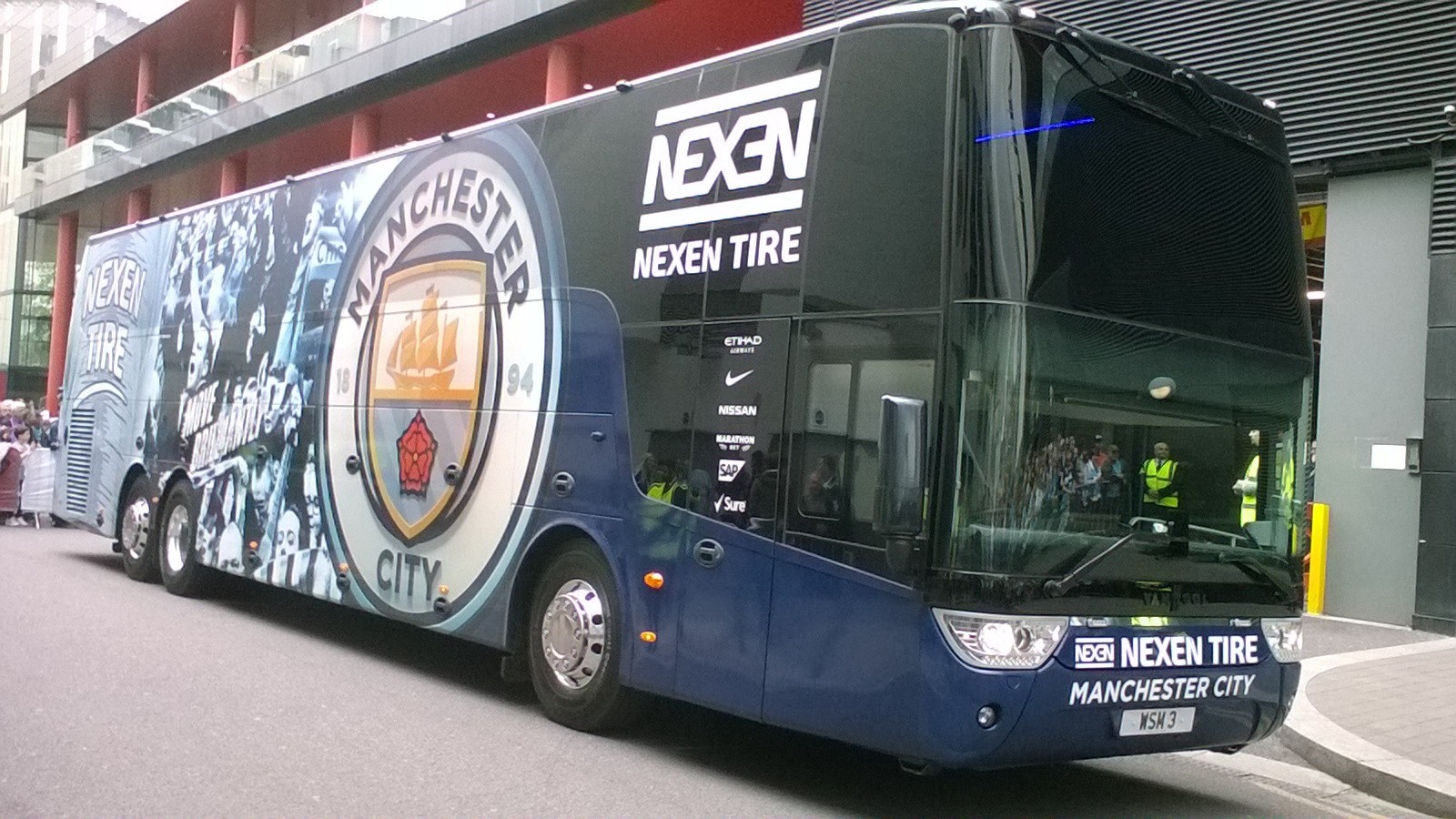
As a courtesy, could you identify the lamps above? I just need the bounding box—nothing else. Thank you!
[1443,105,1456,127]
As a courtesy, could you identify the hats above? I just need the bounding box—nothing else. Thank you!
[1093,434,1103,442]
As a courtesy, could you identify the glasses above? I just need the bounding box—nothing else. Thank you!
[1112,450,1119,454]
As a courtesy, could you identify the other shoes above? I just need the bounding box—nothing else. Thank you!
[5,517,28,527]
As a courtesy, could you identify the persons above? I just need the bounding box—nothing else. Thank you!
[1230,430,1297,557]
[800,456,843,518]
[1080,434,1125,515]
[745,453,792,518]
[0,393,73,528]
[1136,441,1184,535]
[634,450,713,517]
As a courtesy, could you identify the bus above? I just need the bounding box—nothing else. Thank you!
[50,0,1333,777]
